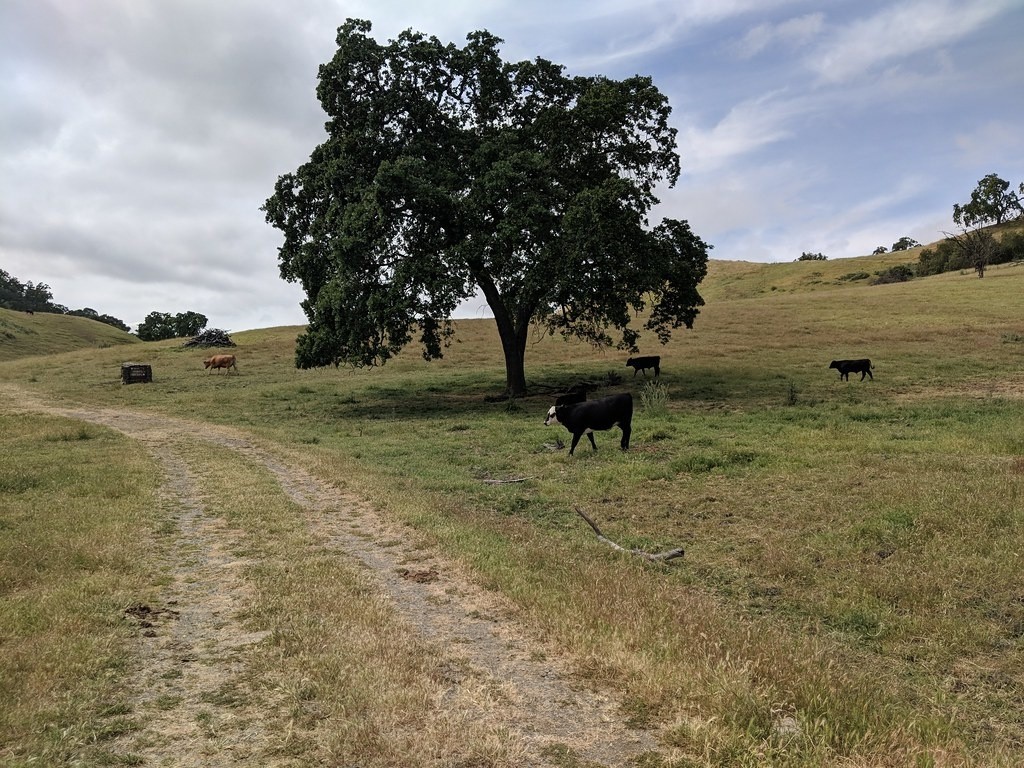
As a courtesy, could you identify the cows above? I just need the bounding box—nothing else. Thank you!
[626,355,661,377]
[544,392,634,456]
[204,355,239,375]
[829,358,876,381]
